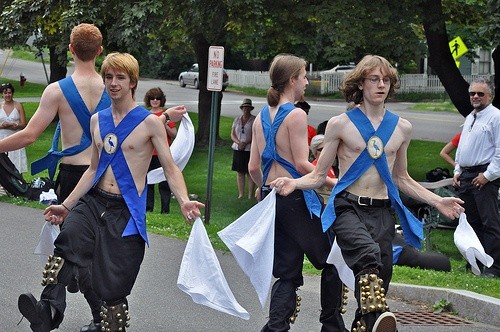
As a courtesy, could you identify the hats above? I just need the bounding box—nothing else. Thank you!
[240,98,254,110]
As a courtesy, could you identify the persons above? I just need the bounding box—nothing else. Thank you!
[270,55,464,332]
[294,100,339,210]
[144,87,177,213]
[18,53,205,332]
[0,82,28,174]
[248,54,349,332]
[0,23,187,332]
[440,132,461,167]
[440,77,500,278]
[231,99,256,200]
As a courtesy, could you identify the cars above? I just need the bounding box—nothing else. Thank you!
[317,65,355,79]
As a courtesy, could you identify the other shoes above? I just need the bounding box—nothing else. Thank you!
[17,292,54,332]
[362,311,397,332]
[80,320,103,332]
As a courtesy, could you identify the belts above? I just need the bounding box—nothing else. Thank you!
[339,190,392,207]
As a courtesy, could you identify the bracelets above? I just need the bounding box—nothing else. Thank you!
[16,122,19,126]
[61,203,71,212]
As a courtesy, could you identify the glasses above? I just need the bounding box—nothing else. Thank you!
[317,147,323,152]
[149,96,162,100]
[1,82,10,87]
[362,75,391,85]
[469,91,486,97]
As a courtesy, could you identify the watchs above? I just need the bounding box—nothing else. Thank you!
[162,112,169,123]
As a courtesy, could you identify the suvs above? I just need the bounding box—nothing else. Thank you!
[179,63,230,92]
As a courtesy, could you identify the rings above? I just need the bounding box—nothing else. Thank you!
[476,184,479,187]
